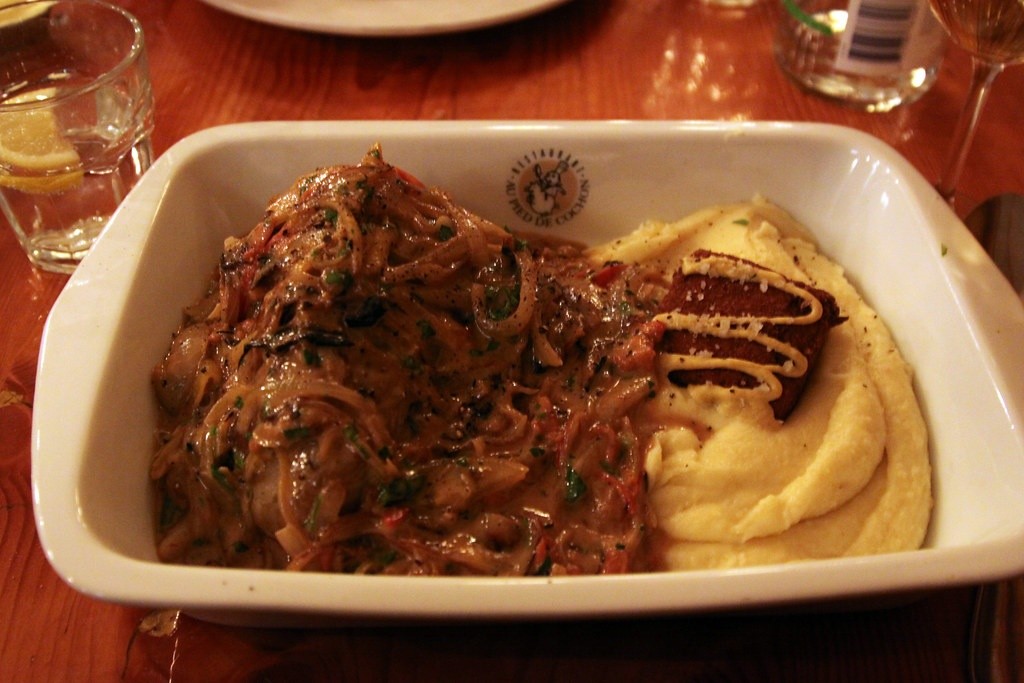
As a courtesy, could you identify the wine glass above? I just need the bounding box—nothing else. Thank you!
[924,0,1023,230]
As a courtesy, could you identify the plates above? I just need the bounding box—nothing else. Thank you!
[202,0,566,36]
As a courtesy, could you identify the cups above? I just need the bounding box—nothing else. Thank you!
[1,0,155,277]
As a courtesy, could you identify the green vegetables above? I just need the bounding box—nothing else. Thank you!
[158,180,586,576]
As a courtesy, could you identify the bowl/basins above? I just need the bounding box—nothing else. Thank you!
[30,124,1024,621]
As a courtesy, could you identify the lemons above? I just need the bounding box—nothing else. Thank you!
[0,87,80,168]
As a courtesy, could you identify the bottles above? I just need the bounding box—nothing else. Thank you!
[782,0,944,113]
